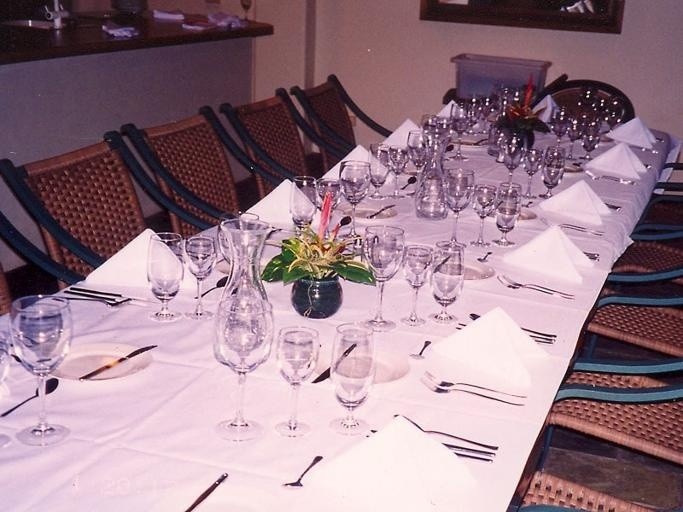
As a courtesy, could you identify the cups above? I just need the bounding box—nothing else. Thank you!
[219,212,259,264]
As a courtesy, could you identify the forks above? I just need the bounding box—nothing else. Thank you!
[457,312,559,346]
[496,275,577,300]
[61,297,131,308]
[421,371,525,405]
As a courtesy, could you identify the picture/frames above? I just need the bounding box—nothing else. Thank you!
[420,1,624,34]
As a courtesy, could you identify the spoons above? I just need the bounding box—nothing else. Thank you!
[285,455,323,488]
[1,378,58,417]
[411,340,431,359]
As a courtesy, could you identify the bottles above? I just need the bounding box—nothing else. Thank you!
[217,218,274,371]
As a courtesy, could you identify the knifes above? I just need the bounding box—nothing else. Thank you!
[312,343,355,384]
[366,427,496,463]
[79,344,156,381]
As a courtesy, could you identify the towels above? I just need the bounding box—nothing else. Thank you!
[436,99,465,118]
[605,116,657,150]
[383,118,437,149]
[585,142,647,180]
[325,416,467,489]
[432,308,547,385]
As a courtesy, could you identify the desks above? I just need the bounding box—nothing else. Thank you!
[1,12,274,301]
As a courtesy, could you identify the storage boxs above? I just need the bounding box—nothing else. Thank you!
[452,52,552,101]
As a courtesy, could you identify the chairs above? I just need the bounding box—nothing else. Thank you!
[530,80,634,124]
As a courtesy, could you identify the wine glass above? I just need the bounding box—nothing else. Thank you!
[185,235,216,321]
[241,0,251,22]
[290,86,622,249]
[430,241,466,325]
[330,323,374,437]
[216,299,275,441]
[358,226,406,331]
[398,245,433,327]
[272,327,320,437]
[10,294,74,447]
[147,232,186,322]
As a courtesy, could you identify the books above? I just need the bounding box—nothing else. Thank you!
[153,8,184,20]
[182,19,215,30]
